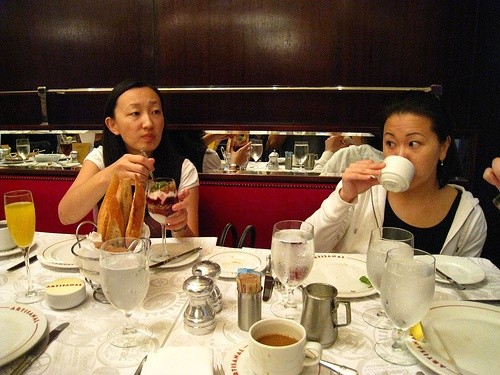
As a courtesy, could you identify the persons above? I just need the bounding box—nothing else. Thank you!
[313,134,383,174]
[301,99,488,258]
[185,129,253,174]
[58,80,200,237]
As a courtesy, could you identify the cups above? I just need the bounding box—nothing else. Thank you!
[301,283,352,350]
[237,286,263,331]
[80,133,95,151]
[376,155,416,193]
[72,143,90,166]
[248,317,323,375]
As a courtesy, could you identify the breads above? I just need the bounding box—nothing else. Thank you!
[93,172,146,252]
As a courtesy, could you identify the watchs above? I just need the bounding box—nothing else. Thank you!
[230,164,240,170]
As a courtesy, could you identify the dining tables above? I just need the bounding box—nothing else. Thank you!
[0,232,499,375]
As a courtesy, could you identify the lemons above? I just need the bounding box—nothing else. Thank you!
[411,322,426,342]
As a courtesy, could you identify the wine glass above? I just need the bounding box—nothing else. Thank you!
[271,218,436,366]
[98,237,153,348]
[233,137,310,174]
[146,177,178,263]
[60,136,77,166]
[3,190,45,304]
[16,138,31,166]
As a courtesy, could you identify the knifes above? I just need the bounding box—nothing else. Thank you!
[6,254,38,272]
[9,322,70,375]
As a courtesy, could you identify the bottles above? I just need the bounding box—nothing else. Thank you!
[180,260,224,336]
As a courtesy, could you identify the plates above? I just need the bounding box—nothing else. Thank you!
[224,341,321,375]
[0,234,265,280]
[296,252,379,298]
[0,302,48,369]
[404,300,500,375]
[415,254,485,285]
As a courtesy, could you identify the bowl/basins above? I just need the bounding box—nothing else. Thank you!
[34,154,60,162]
[0,220,17,251]
[47,277,87,310]
